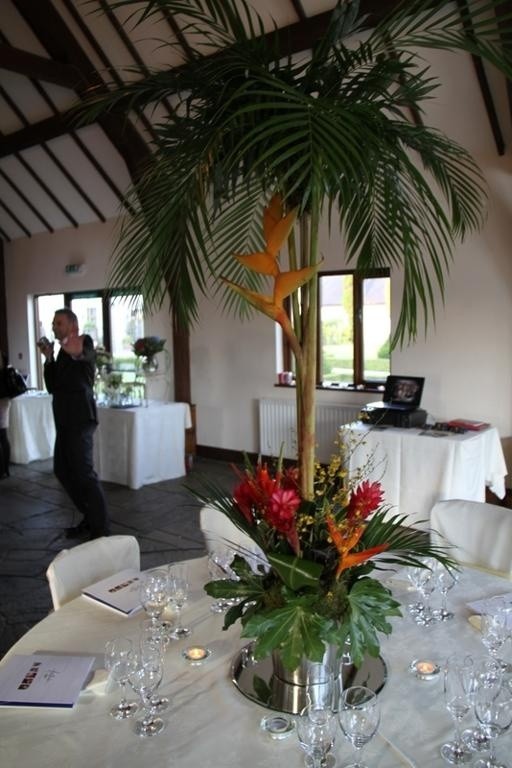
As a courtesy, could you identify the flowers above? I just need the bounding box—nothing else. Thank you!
[180,422,460,659]
[132,334,167,369]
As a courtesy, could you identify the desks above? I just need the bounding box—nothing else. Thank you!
[338,418,496,529]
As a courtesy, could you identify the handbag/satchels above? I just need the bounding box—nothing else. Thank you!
[3,363,28,398]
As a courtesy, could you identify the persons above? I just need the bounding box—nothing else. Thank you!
[1,347,19,483]
[35,306,110,543]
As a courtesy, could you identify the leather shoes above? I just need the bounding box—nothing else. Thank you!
[67,520,110,541]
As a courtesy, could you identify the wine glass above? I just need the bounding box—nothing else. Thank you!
[407,556,512,767]
[98,561,191,738]
[206,550,262,614]
[295,659,381,767]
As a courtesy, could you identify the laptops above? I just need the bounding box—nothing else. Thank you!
[367,376,425,412]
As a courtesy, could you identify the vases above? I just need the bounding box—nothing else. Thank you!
[142,353,158,373]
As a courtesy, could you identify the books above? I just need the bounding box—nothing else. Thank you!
[392,556,436,586]
[465,591,511,626]
[79,565,159,617]
[447,418,490,433]
[0,649,98,710]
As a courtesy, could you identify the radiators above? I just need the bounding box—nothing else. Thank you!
[258,397,364,470]
